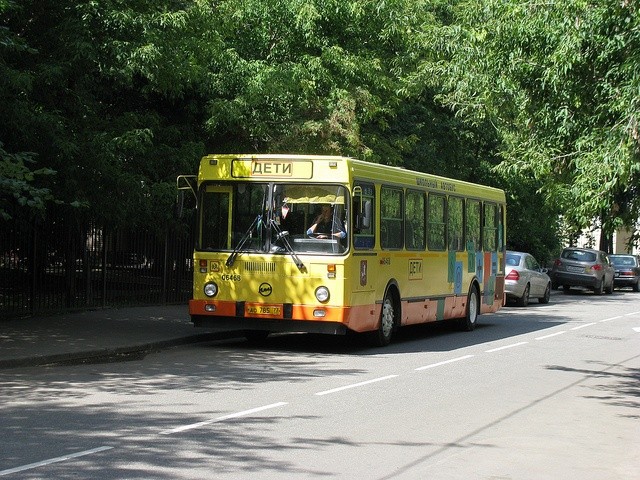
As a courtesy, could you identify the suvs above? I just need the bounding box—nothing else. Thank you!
[552,247,615,295]
[607,254,640,291]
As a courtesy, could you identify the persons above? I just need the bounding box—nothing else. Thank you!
[307,205,346,239]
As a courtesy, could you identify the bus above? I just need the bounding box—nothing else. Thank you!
[176,153,507,345]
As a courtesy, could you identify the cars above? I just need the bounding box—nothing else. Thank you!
[506,251,553,307]
